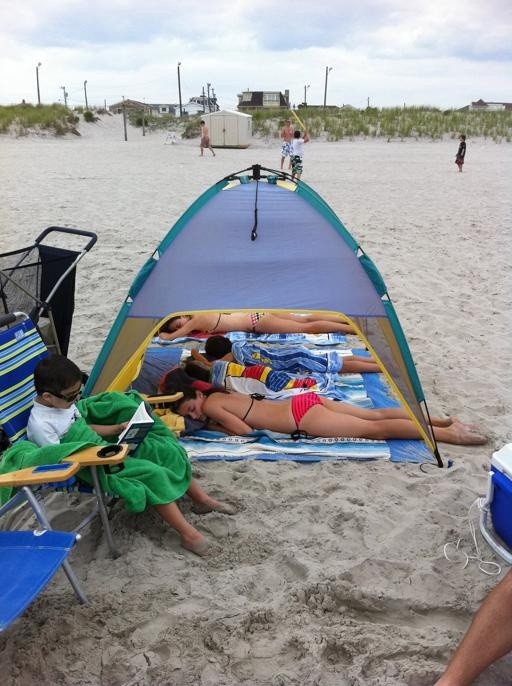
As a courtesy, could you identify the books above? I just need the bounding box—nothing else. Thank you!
[116,400,154,456]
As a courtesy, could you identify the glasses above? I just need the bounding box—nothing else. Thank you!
[48,390,82,402]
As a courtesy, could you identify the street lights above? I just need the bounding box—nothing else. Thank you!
[60,86,68,105]
[177,62,183,114]
[34,62,43,106]
[84,79,89,109]
[305,84,310,104]
[324,66,332,107]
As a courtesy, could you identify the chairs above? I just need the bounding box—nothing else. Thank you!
[0,461,87,634]
[0,312,185,558]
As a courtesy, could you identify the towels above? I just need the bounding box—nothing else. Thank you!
[209,341,342,400]
[0,389,191,513]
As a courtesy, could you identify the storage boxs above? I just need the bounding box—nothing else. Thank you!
[479,440,512,565]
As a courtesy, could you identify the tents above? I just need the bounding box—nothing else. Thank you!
[76,163,443,468]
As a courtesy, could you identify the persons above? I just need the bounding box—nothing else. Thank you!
[280,119,293,170]
[200,119,215,157]
[191,334,383,374]
[160,384,488,447]
[26,355,239,558]
[154,311,357,341]
[454,134,467,172]
[291,130,310,180]
[183,357,317,392]
[432,567,512,686]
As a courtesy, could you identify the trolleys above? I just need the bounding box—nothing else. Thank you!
[0,225,97,358]
